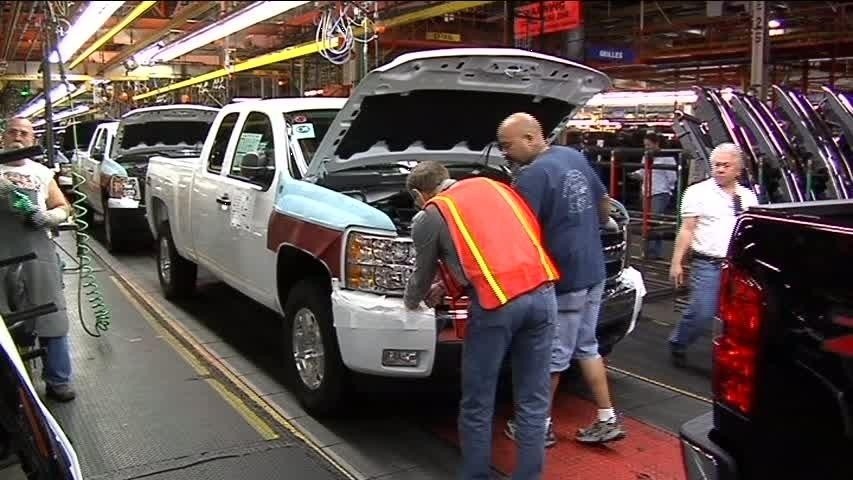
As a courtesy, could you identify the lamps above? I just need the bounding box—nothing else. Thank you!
[12,0,310,127]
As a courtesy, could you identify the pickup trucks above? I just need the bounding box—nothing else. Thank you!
[72,103,223,254]
[680,201,852,480]
[145,48,647,417]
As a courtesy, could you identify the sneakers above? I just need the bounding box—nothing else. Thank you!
[574,419,627,442]
[502,416,557,448]
[45,383,76,402]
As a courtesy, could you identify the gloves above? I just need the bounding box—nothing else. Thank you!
[32,208,68,226]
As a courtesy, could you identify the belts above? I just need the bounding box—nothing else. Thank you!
[692,249,727,265]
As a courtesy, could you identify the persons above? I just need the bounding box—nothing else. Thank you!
[405,159,562,480]
[665,142,758,366]
[0,115,76,402]
[627,134,679,262]
[496,112,624,449]
